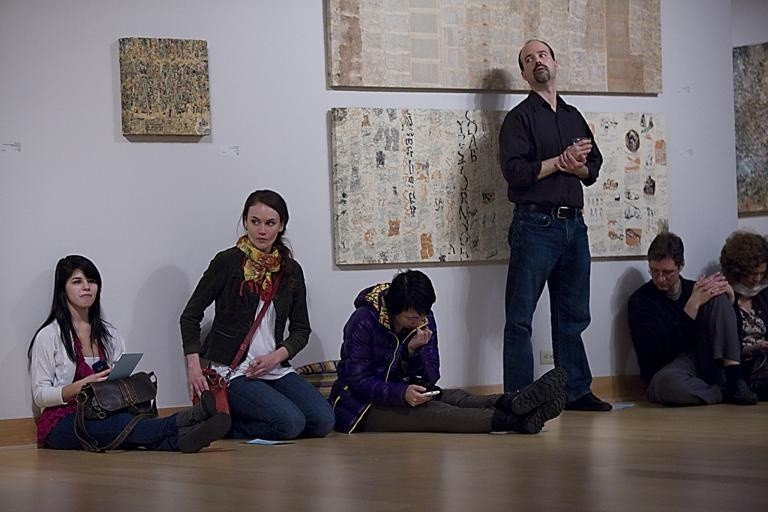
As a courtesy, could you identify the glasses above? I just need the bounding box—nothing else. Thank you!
[647,268,679,278]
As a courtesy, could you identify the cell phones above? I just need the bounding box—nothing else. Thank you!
[92,360,110,377]
[422,390,440,397]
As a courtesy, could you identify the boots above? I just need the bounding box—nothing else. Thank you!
[492,391,565,434]
[493,367,568,413]
[178,412,232,454]
[177,390,218,425]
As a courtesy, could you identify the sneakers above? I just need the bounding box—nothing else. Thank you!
[725,381,758,406]
[568,393,613,412]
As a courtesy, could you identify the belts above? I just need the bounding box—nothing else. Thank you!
[533,204,580,219]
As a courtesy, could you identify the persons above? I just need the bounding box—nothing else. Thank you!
[721,230,768,399]
[498,40,615,412]
[625,232,758,406]
[326,270,569,436]
[29,254,231,451]
[181,187,337,438]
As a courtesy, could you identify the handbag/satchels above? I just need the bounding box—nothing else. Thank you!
[192,368,231,415]
[78,370,159,419]
